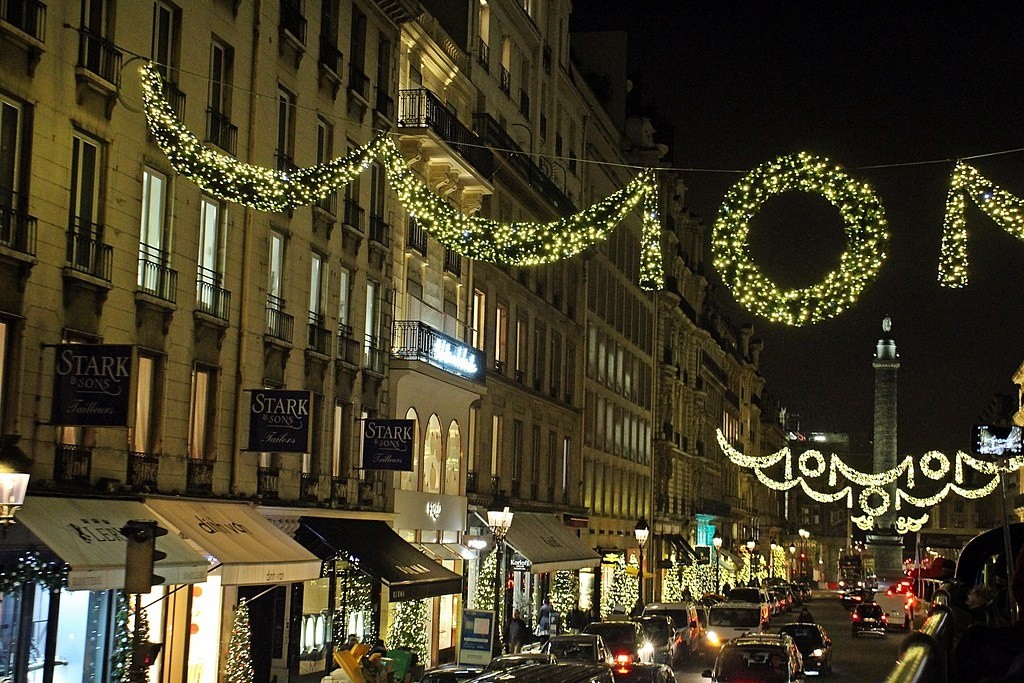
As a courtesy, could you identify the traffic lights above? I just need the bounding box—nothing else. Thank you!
[124,519,168,594]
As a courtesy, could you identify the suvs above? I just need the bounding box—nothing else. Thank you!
[701,631,803,683]
[852,603,888,639]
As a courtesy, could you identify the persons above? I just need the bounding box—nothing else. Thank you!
[507,609,526,654]
[335,634,420,683]
[630,599,646,620]
[798,607,814,623]
[683,574,783,608]
[535,598,601,651]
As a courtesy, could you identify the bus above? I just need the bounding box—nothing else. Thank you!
[838,557,878,592]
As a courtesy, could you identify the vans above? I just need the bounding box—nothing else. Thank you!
[640,601,701,660]
[706,602,766,664]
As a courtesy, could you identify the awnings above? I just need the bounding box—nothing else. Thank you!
[298,516,465,602]
[473,510,602,574]
[11,495,211,591]
[144,498,323,586]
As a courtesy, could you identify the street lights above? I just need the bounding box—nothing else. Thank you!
[789,543,796,584]
[485,494,516,658]
[745,535,756,587]
[712,532,723,595]
[799,528,810,584]
[769,538,778,577]
[634,517,651,615]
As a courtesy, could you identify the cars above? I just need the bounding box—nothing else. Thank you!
[614,662,678,683]
[778,623,833,677]
[583,620,654,674]
[632,616,684,671]
[539,633,614,664]
[416,654,614,683]
[695,579,812,624]
[840,589,875,609]
